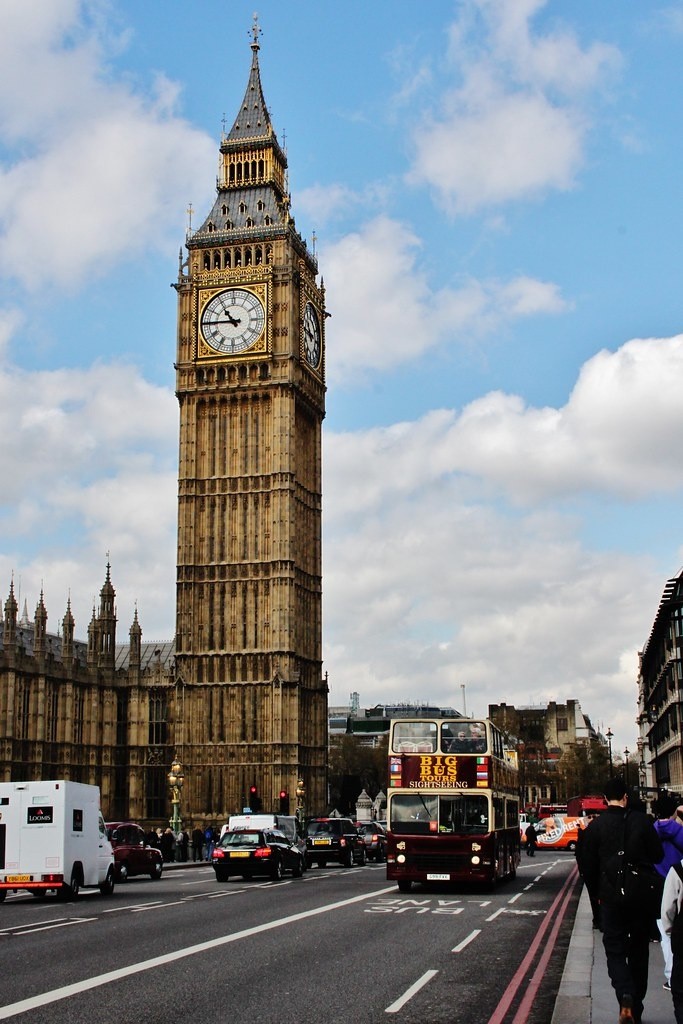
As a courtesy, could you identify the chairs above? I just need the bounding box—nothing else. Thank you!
[396,741,433,752]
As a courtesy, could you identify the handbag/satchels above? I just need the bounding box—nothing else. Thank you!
[616,861,664,920]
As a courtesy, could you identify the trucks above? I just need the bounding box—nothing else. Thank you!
[0,779,119,901]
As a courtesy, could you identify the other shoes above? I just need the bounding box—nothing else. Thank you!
[618,1006,635,1024]
[663,982,671,991]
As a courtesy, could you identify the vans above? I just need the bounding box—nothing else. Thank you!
[220,814,312,870]
[519,813,593,851]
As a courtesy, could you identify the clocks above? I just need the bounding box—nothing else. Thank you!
[303,301,322,369]
[198,287,265,354]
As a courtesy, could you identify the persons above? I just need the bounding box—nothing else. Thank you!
[146,823,221,863]
[574,824,582,856]
[449,732,469,753]
[525,822,537,857]
[574,781,683,1024]
[467,727,486,752]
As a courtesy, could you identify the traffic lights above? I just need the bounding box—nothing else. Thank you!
[249,786,260,811]
[280,791,290,815]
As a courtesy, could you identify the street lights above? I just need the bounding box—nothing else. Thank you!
[606,727,615,779]
[167,753,185,862]
[295,774,306,833]
[623,746,631,790]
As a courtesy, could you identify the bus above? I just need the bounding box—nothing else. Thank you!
[539,804,569,819]
[566,795,607,818]
[384,717,522,892]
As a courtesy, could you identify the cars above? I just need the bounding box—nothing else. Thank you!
[305,818,388,868]
[104,822,164,882]
[212,828,304,883]
